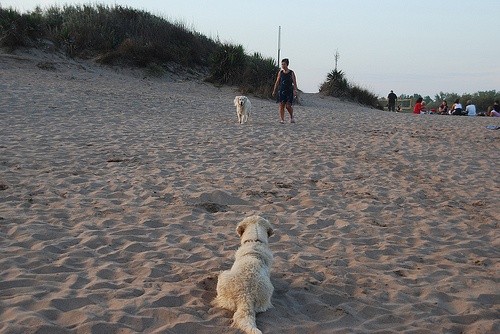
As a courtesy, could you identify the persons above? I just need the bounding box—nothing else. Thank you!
[376,104,383,110]
[387,90,397,112]
[271,59,297,123]
[413,97,500,117]
[397,105,402,112]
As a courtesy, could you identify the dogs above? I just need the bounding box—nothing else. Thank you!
[233,96,252,124]
[210,215,276,334]
